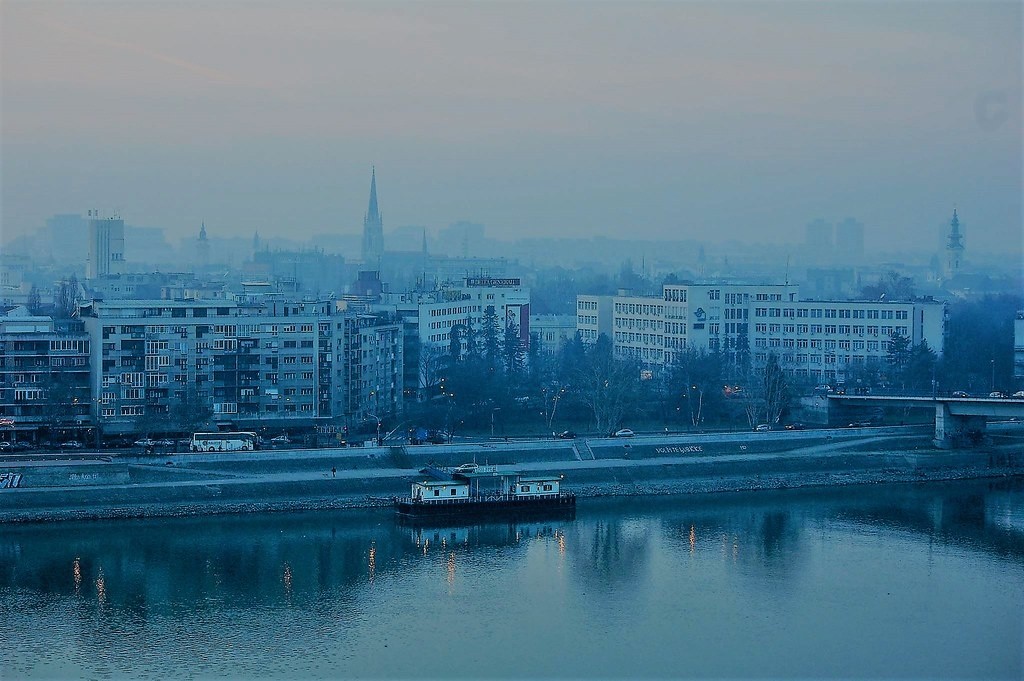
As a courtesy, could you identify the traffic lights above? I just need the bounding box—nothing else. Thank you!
[379,422,382,429]
[344,427,347,430]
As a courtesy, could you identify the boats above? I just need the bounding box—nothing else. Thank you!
[393,469,577,519]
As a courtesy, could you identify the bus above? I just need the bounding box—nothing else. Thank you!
[193,431,259,450]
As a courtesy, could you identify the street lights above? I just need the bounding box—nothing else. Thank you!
[93,398,101,420]
[492,407,501,438]
[992,360,995,390]
[279,396,290,447]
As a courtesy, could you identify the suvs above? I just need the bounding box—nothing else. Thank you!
[61,440,82,449]
[109,438,134,449]
[87,439,109,448]
[135,438,155,448]
[0,442,11,450]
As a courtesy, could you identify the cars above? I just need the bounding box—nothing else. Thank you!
[1012,391,1024,398]
[953,391,970,398]
[815,384,831,392]
[989,390,1004,399]
[752,424,772,432]
[180,438,191,445]
[410,432,449,444]
[270,436,292,444]
[156,439,174,447]
[611,429,634,437]
[557,431,578,440]
[785,424,805,429]
[836,387,845,394]
